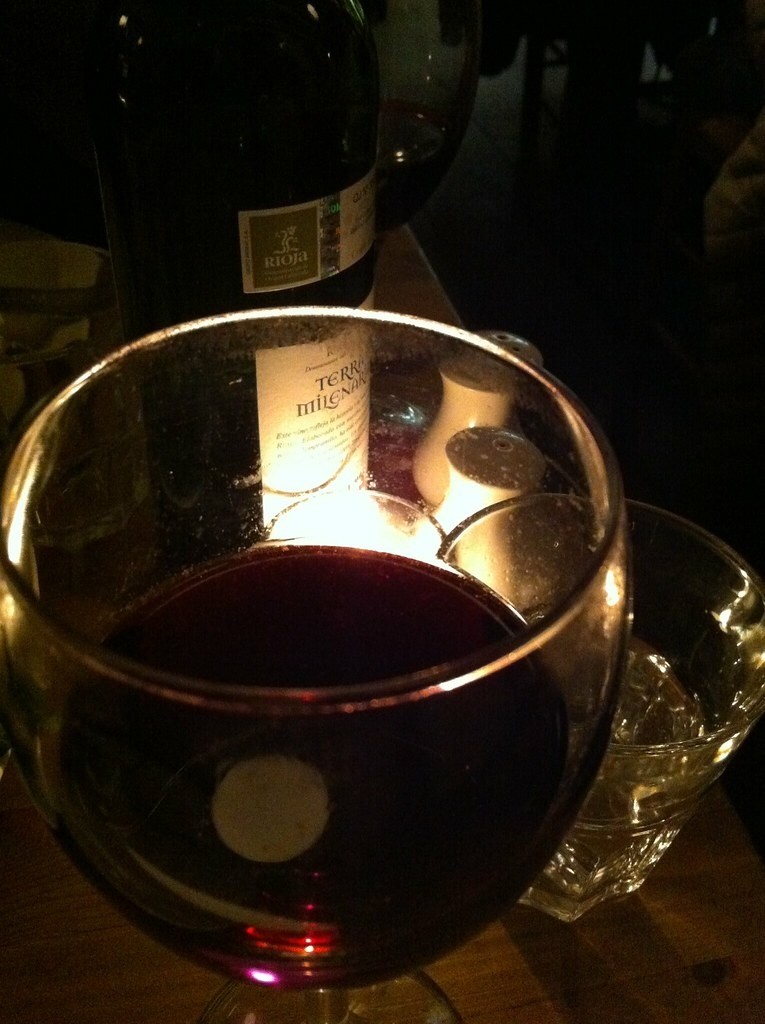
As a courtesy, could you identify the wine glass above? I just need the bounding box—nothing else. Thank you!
[2,303,635,1024]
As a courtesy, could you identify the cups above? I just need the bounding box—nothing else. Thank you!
[434,490,765,922]
[360,0,486,236]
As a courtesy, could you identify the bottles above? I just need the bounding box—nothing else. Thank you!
[99,0,385,552]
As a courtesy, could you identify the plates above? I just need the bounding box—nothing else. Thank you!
[2,242,112,367]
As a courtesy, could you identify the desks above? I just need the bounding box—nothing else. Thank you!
[0,223,765,1024]
